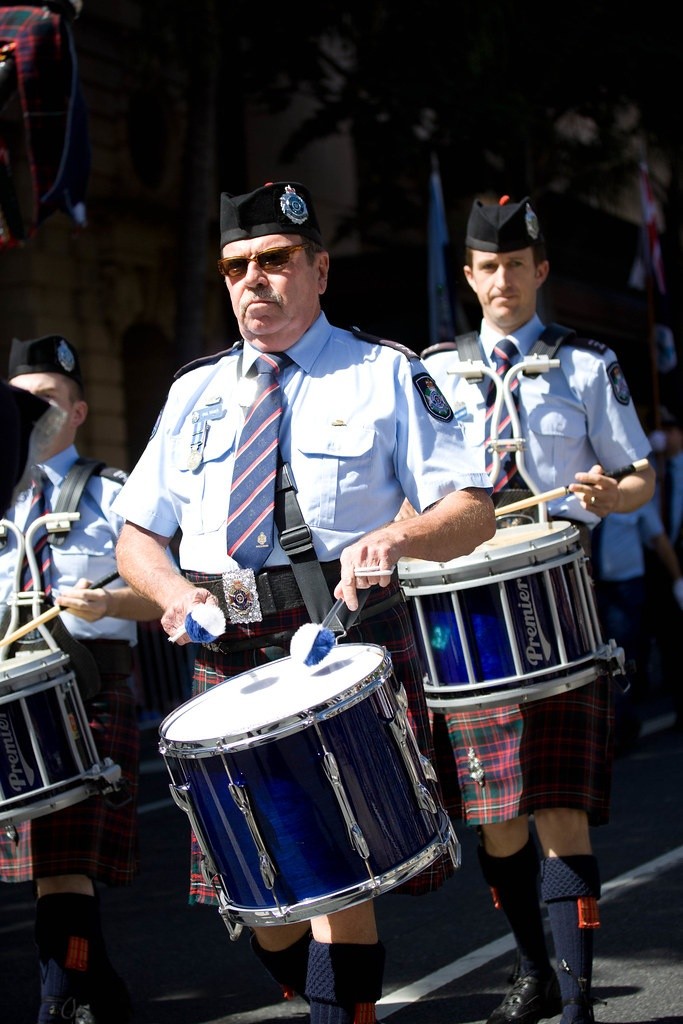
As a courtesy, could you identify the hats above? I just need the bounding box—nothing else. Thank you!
[220,182,323,248]
[7,335,82,386]
[465,196,539,253]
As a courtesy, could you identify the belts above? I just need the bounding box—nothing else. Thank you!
[195,560,398,625]
[490,489,539,528]
[0,603,73,658]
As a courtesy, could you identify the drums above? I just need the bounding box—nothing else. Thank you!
[0,647,126,828]
[397,519,614,712]
[155,635,460,929]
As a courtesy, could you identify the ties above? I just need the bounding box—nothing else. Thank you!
[226,353,294,577]
[21,465,51,602]
[485,339,520,492]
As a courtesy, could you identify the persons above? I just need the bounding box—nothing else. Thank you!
[0,332,162,1023]
[109,179,497,1023]
[598,396,682,751]
[421,193,655,1022]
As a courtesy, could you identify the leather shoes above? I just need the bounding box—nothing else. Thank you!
[486,967,563,1024]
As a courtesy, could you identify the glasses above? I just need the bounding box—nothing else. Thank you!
[217,243,314,277]
